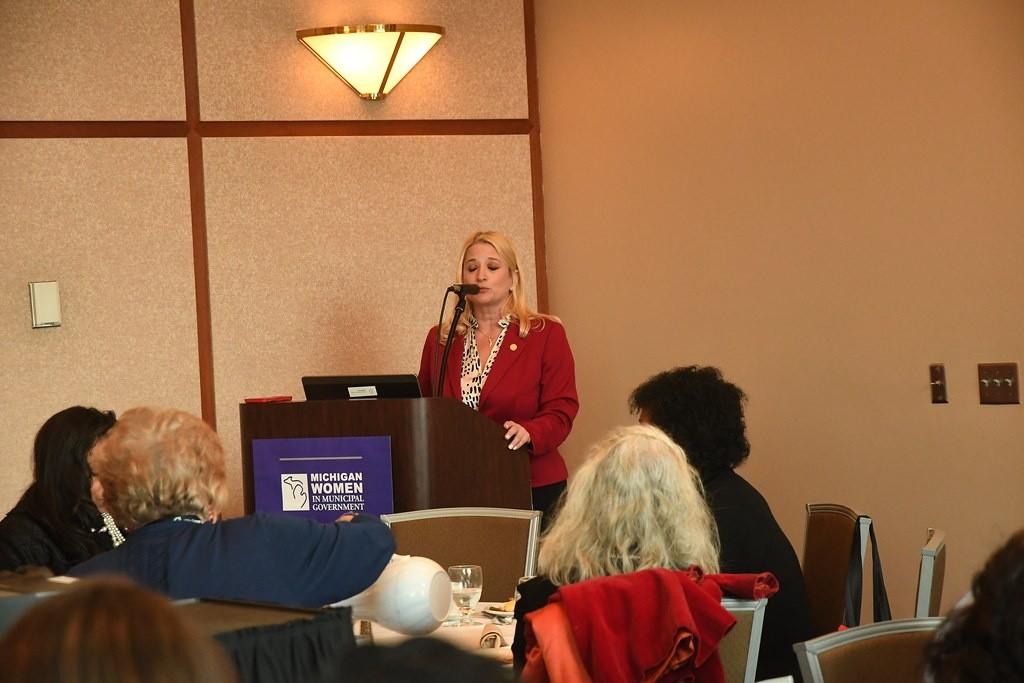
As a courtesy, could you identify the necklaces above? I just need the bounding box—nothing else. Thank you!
[478,328,502,347]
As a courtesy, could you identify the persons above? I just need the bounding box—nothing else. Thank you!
[0,406,130,578]
[417,230,579,534]
[63,406,397,608]
[628,365,817,683]
[511,422,721,683]
[0,575,237,683]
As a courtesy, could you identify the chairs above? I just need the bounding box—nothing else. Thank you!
[368,501,965,683]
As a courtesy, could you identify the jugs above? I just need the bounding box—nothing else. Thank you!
[329,550,452,638]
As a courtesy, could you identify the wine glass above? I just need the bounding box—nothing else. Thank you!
[448,564,483,628]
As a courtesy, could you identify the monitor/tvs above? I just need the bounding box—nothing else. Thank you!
[302,374,423,401]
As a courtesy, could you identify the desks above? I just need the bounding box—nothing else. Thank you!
[351,601,524,664]
[0,568,342,648]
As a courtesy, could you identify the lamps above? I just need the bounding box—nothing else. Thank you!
[297,24,446,102]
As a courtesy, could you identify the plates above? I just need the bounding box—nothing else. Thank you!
[484,604,514,618]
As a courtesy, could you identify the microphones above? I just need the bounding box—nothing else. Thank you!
[447,284,480,295]
